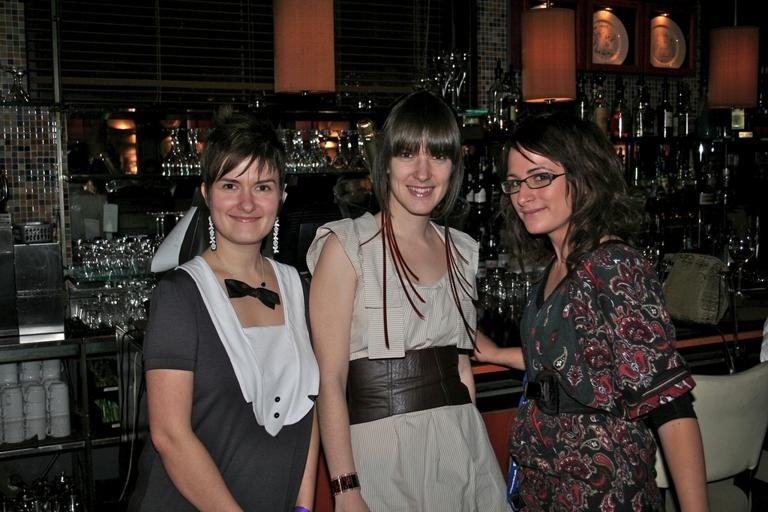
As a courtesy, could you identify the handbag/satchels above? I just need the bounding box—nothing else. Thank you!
[661,252,730,325]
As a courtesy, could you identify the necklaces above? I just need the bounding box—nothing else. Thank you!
[213,244,281,309]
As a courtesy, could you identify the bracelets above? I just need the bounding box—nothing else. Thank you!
[329,472,361,500]
[292,503,314,511]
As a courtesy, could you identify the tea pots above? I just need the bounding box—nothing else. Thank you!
[1,471,82,511]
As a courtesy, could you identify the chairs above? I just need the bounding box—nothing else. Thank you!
[654,360,768,511]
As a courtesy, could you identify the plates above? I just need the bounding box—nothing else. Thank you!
[592,10,629,65]
[650,16,687,69]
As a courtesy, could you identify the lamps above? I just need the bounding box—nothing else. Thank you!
[520,1,577,105]
[706,0,759,108]
[273,0,336,96]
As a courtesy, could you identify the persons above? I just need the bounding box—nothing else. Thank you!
[122,111,324,510]
[305,85,516,511]
[474,104,714,512]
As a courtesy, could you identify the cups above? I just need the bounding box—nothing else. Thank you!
[72,233,160,330]
[0,358,72,444]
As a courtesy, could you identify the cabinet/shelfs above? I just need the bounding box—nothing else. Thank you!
[575,0,698,78]
[0,330,153,512]
[68,88,768,279]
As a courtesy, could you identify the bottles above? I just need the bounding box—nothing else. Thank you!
[474,230,511,279]
[618,143,733,209]
[577,76,690,138]
[463,156,501,216]
[488,60,522,131]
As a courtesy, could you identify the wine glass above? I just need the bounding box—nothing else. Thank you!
[728,234,755,299]
[276,129,369,174]
[161,127,202,177]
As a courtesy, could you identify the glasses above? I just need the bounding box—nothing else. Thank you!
[500,171,566,195]
